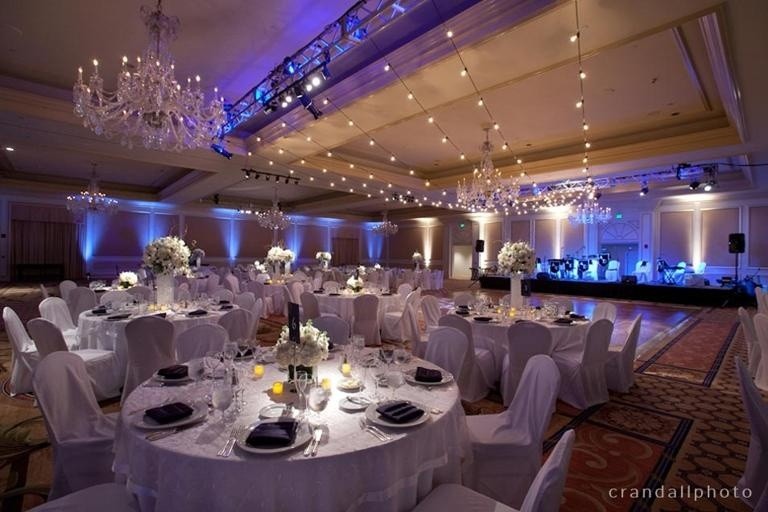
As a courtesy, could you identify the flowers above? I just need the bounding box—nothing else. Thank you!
[315,251,332,261]
[266,245,295,264]
[272,317,331,368]
[497,240,536,275]
[144,235,194,279]
[412,252,422,262]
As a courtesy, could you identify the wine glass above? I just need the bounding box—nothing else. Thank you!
[308,383,328,425]
[351,335,366,357]
[204,337,250,388]
[211,383,234,423]
[369,350,408,402]
[295,371,306,411]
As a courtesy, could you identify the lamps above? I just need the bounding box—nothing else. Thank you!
[454,126,521,209]
[63,163,120,218]
[507,180,714,207]
[256,183,294,231]
[565,180,613,225]
[371,211,399,235]
[390,191,419,205]
[72,0,228,154]
[210,42,332,185]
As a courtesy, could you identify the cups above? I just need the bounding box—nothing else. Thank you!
[342,364,350,375]
[273,382,283,397]
[322,378,331,392]
[254,363,264,379]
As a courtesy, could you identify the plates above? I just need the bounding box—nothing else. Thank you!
[339,377,362,390]
[259,403,290,417]
[553,319,577,326]
[154,369,194,385]
[339,395,367,412]
[405,366,454,385]
[237,417,314,454]
[366,400,429,427]
[134,400,207,428]
[473,315,490,323]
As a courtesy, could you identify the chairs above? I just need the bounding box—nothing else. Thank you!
[533,256,707,288]
[734,286,768,512]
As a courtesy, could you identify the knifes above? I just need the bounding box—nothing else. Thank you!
[313,428,324,456]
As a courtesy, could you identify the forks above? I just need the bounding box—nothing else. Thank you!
[218,425,244,457]
